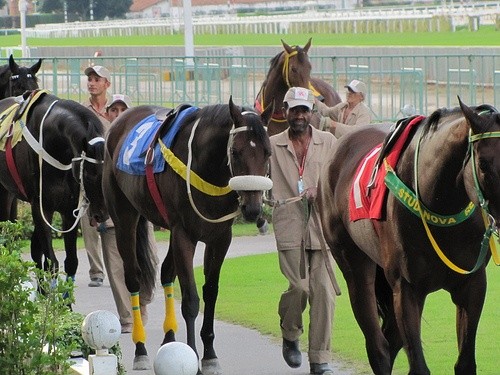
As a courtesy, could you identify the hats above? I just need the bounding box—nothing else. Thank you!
[344,80,368,95]
[284,87,315,110]
[105,94,131,109]
[85,66,111,81]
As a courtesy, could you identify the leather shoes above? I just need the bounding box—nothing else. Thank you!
[310,363,334,375]
[283,337,302,368]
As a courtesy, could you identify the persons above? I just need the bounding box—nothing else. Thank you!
[89,52,102,68]
[81,66,112,287]
[237,87,341,375]
[312,80,371,139]
[84,94,159,334]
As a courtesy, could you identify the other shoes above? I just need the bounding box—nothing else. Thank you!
[121,323,133,333]
[139,304,148,327]
[88,278,103,287]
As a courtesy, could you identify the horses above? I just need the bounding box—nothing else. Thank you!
[103,104,271,375]
[0,89,109,313]
[312,93,499,375]
[0,54,42,101]
[253,37,342,182]
